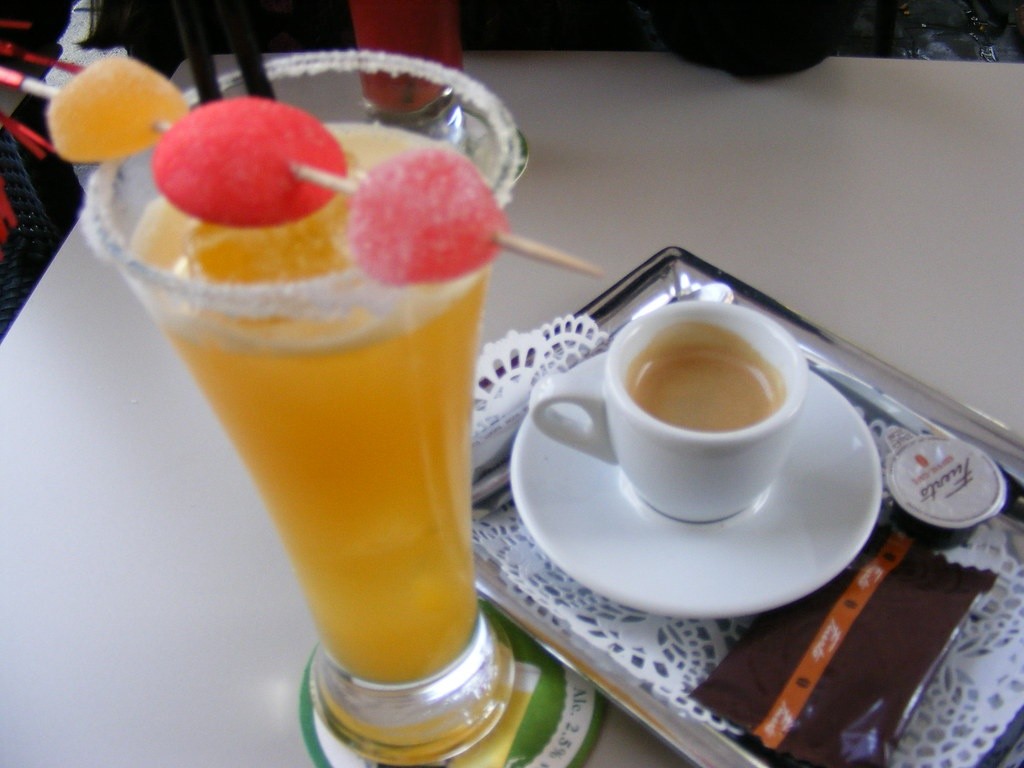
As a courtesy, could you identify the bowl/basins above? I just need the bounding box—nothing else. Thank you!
[885,425,1007,550]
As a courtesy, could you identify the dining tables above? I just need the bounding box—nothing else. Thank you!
[0,39,1024,768]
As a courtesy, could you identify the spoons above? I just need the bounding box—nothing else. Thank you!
[470,282,734,478]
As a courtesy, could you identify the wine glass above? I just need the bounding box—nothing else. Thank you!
[80,48,515,763]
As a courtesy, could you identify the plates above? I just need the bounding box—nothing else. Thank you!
[511,353,882,619]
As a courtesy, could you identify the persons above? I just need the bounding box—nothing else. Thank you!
[0,0,160,347]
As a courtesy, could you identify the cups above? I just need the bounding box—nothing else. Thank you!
[350,0,463,147]
[528,302,810,524]
